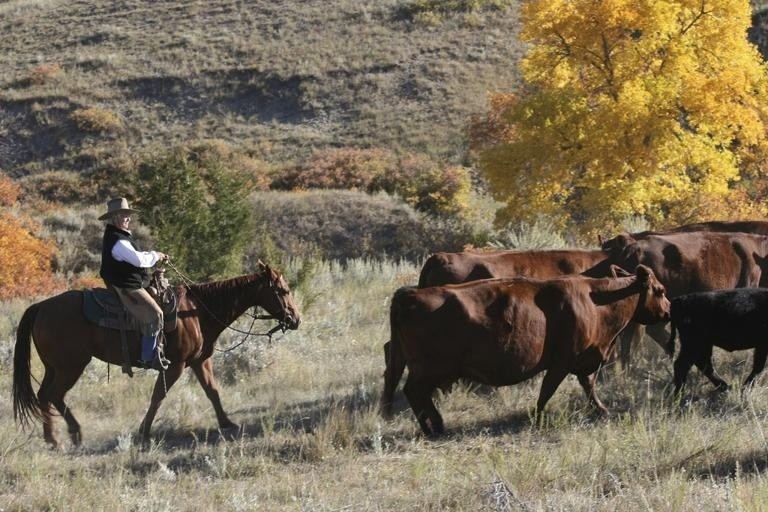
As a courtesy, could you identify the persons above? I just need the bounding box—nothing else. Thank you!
[99,197,170,370]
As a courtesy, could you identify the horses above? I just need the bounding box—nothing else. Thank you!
[11,257,304,453]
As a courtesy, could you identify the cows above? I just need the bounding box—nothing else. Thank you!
[376,217,768,438]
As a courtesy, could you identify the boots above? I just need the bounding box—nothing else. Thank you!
[142,335,171,369]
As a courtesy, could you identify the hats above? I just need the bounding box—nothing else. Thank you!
[97,197,141,220]
